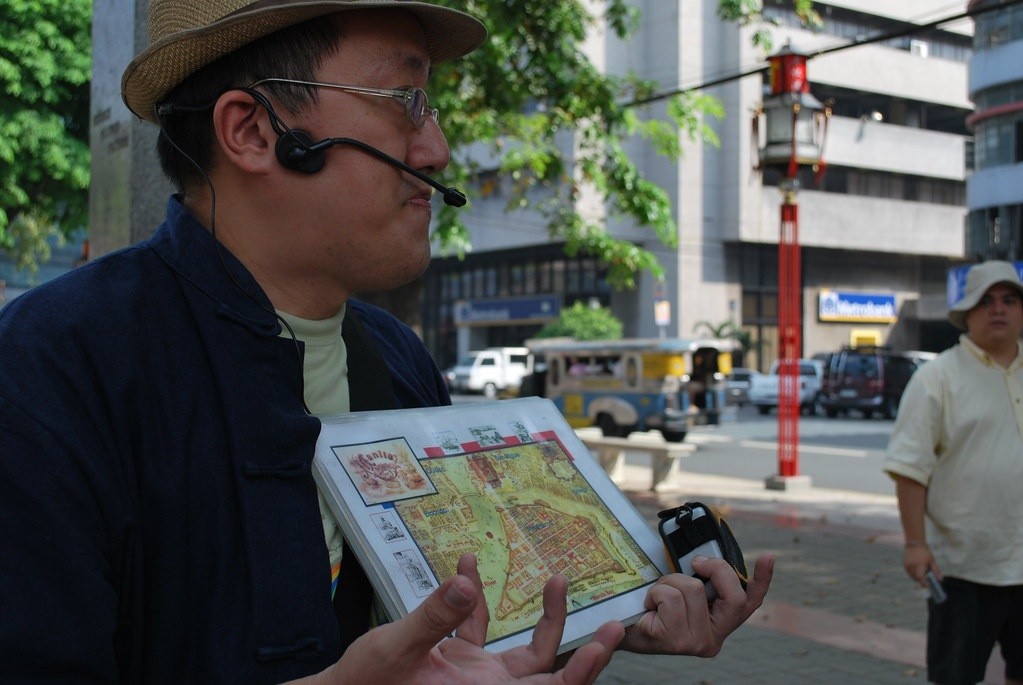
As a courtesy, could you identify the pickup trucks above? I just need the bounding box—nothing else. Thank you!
[442,346,535,399]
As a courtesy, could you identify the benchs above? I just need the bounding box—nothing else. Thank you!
[569,426,697,490]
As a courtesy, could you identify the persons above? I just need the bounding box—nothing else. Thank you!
[882,260,1023,684]
[597,361,613,376]
[0,0,777,685]
[568,357,585,374]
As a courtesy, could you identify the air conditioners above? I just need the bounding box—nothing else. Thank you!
[910,40,928,57]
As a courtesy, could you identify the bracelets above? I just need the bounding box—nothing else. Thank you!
[905,541,925,546]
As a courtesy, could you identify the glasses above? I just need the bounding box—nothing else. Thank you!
[248,78,440,130]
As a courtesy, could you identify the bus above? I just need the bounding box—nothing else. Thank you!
[519,336,752,443]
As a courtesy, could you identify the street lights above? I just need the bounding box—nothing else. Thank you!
[748,37,838,491]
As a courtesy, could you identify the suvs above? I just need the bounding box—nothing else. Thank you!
[726,344,944,421]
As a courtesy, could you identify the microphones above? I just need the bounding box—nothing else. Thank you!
[275,130,468,207]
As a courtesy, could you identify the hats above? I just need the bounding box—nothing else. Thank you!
[120,0,488,125]
[947,259,1023,329]
